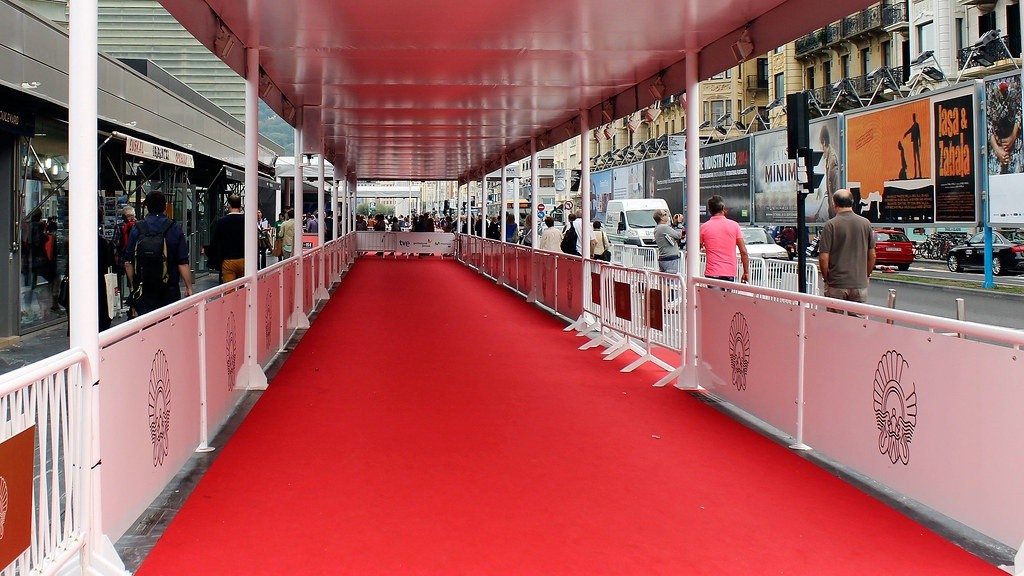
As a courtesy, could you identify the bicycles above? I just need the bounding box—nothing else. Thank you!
[913,231,973,261]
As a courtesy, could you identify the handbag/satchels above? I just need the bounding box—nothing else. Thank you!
[272,240,282,257]
[104,266,122,319]
[59,279,68,306]
[519,236,525,245]
[603,250,611,262]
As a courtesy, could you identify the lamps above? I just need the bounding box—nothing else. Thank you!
[260,78,272,97]
[717,114,741,136]
[741,104,768,130]
[645,101,662,122]
[907,66,943,97]
[911,50,950,89]
[730,34,754,63]
[601,103,613,121]
[893,72,893,73]
[284,101,296,120]
[539,135,548,147]
[679,93,688,109]
[564,127,575,137]
[215,35,234,57]
[604,122,617,140]
[802,89,824,117]
[578,128,687,172]
[765,98,787,117]
[828,82,845,116]
[648,77,665,99]
[723,121,745,141]
[867,67,904,98]
[771,105,787,130]
[868,77,900,106]
[833,78,864,108]
[628,112,641,132]
[699,120,721,142]
[953,50,994,85]
[595,126,605,143]
[975,30,1019,70]
[705,125,726,145]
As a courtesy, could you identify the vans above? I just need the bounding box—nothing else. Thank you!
[604,199,679,261]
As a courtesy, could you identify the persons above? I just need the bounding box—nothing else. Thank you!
[819,126,839,218]
[59,191,193,337]
[257,206,353,270]
[21,206,63,316]
[653,209,682,314]
[700,196,749,293]
[987,83,1022,167]
[819,188,876,319]
[213,194,245,294]
[771,226,798,248]
[356,207,610,260]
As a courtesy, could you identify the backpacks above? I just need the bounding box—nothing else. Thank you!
[561,221,577,253]
[133,220,173,287]
[119,222,136,251]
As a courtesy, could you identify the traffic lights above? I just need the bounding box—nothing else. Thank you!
[58,196,69,230]
[444,200,450,210]
[805,148,824,194]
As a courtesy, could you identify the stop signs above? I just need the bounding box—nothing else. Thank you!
[537,203,545,212]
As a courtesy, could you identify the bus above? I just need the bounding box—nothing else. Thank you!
[488,198,532,233]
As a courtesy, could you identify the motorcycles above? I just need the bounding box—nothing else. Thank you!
[778,234,796,262]
[805,236,821,258]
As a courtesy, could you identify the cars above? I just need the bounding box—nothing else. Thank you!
[947,229,1024,277]
[736,226,789,278]
[538,222,564,235]
[871,228,914,272]
